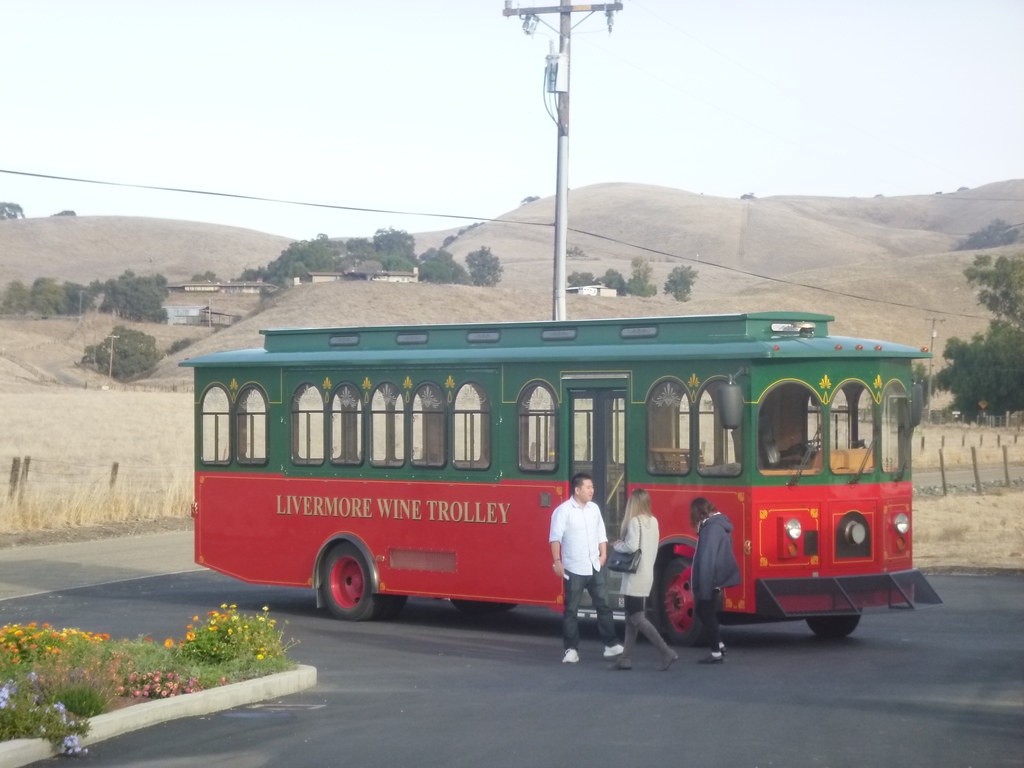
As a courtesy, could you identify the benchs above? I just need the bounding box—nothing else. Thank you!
[650,448,705,475]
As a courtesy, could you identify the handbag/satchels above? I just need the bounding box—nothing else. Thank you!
[607,516,642,573]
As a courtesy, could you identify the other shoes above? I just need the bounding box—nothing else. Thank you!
[655,650,678,670]
[698,655,721,664]
[606,662,630,670]
[721,646,727,656]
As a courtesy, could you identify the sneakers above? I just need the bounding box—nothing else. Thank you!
[562,648,579,663]
[603,644,624,656]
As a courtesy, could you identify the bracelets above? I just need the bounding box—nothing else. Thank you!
[554,559,560,561]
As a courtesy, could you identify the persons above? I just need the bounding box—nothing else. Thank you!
[550,474,623,663]
[761,425,780,467]
[690,496,741,662]
[607,488,678,671]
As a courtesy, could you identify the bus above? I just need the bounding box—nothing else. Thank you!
[178,307,944,651]
